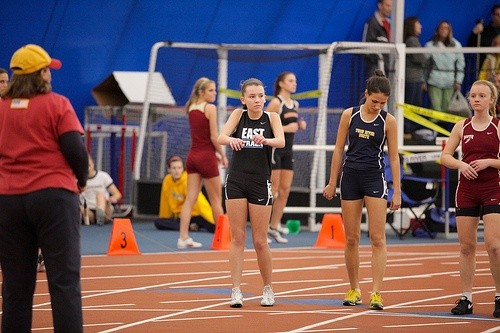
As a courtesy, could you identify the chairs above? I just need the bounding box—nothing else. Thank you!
[384,154,446,241]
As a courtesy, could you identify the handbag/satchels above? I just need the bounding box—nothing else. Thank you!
[448,91,470,117]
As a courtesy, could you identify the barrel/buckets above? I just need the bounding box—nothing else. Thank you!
[286,219,300,234]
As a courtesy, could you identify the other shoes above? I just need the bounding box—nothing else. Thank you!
[96,209,105,225]
[189,223,199,231]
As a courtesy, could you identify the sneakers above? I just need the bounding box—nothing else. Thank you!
[275,226,289,235]
[230,287,243,307]
[178,236,201,249]
[368,292,383,309]
[342,290,362,305]
[267,225,288,243]
[493,296,500,317]
[260,285,275,305]
[451,296,473,314]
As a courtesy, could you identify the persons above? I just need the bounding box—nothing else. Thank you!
[217,79,286,308]
[420,20,465,122]
[0,43,88,333]
[363,0,391,75]
[323,69,401,310]
[480,36,500,121]
[403,16,430,133]
[153,156,216,233]
[266,72,306,243]
[467,3,500,69]
[79,157,121,225]
[0,68,9,93]
[177,77,230,249]
[440,79,500,317]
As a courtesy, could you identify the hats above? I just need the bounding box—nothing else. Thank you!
[10,44,62,75]
